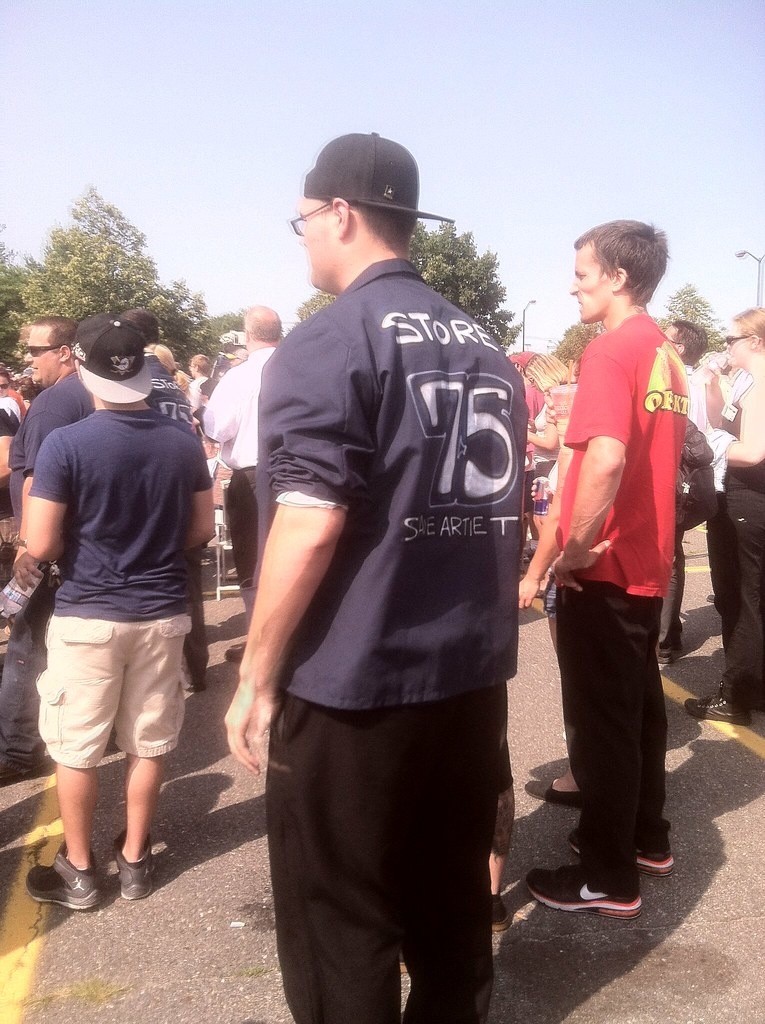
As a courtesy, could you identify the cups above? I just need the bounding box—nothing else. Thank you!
[549,384,577,456]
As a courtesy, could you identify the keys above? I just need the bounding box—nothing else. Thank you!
[51,565,61,585]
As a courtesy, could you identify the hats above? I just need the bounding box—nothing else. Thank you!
[69,311,154,405]
[303,132,458,228]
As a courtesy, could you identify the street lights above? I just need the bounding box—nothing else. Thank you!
[520,299,543,357]
[734,250,764,310]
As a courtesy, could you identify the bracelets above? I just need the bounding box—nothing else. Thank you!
[18,538,27,548]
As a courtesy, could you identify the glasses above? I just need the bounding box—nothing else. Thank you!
[0,383,12,390]
[23,343,67,359]
[727,335,749,343]
[290,199,360,235]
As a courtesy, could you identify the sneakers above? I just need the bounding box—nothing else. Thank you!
[526,863,643,920]
[567,827,675,877]
[25,841,100,910]
[112,826,154,901]
[684,692,754,728]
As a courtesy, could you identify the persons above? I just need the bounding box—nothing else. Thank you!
[222,133,527,1024]
[471,308,765,932]
[526,220,690,919]
[22,314,216,910]
[119,306,283,692]
[0,362,44,635]
[0,316,95,781]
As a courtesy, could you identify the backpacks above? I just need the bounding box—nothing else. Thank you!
[671,417,720,532]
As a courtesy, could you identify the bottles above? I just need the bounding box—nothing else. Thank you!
[1,569,44,619]
[691,351,729,385]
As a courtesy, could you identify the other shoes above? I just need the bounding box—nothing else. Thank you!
[225,646,246,665]
[525,779,584,808]
[657,641,686,664]
[491,894,511,933]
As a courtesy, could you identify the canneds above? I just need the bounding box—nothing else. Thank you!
[533,477,550,515]
